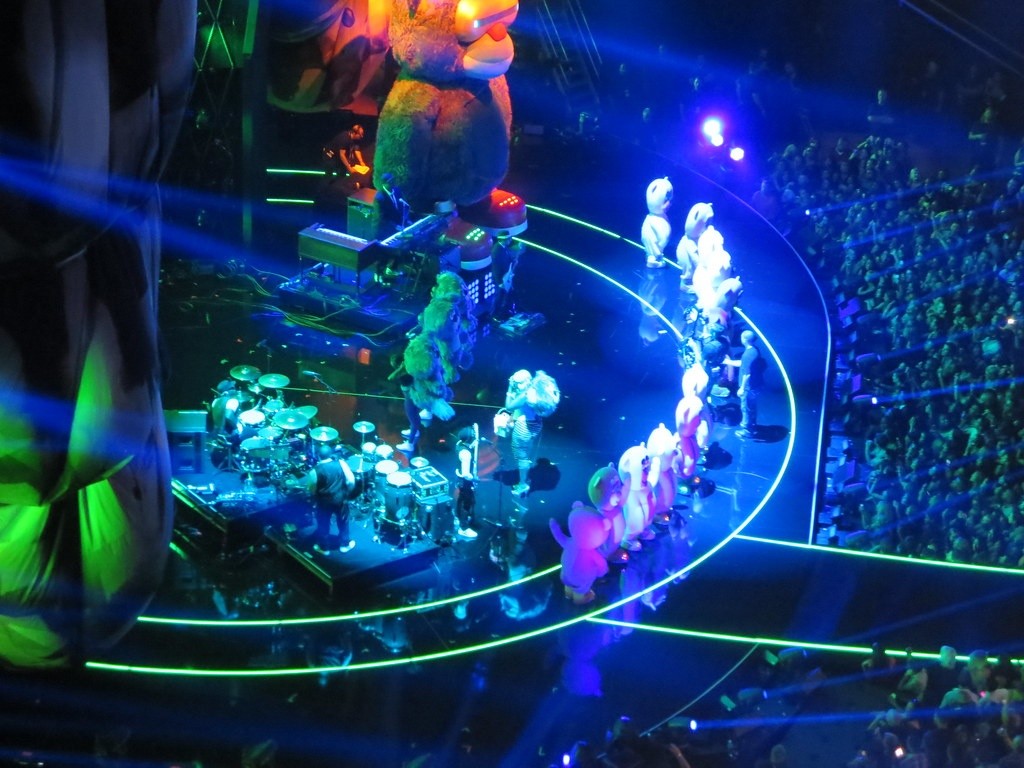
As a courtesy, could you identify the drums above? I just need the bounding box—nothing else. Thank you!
[237,410,266,432]
[345,440,429,522]
[353,420,377,445]
[309,426,339,458]
[258,427,284,445]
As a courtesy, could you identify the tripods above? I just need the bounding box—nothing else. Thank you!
[482,444,524,542]
[407,491,432,545]
[354,458,373,511]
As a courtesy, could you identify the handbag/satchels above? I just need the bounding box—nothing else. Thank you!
[494,413,514,438]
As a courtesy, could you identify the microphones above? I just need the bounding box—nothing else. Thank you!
[302,371,321,376]
[256,338,267,347]
[481,437,493,444]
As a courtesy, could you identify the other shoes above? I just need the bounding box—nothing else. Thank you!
[386,268,403,277]
[513,480,532,489]
[396,440,414,452]
[374,273,392,286]
[340,541,355,553]
[735,429,753,438]
[313,544,330,555]
[454,515,471,525]
[512,498,528,517]
[401,429,419,437]
[458,527,477,537]
[740,421,756,428]
[511,486,530,498]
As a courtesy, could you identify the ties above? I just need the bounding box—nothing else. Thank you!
[391,195,396,207]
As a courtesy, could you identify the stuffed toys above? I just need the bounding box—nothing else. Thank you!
[549,175,745,606]
[374,0,529,271]
[403,269,480,420]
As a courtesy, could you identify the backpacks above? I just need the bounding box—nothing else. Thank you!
[525,371,560,417]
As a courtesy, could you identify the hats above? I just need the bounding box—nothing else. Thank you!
[381,172,394,182]
[457,428,475,443]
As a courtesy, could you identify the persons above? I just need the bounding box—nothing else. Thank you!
[207,379,241,443]
[722,330,762,440]
[390,285,438,452]
[506,370,544,496]
[319,124,365,197]
[560,40,1023,768]
[309,445,356,556]
[453,427,481,540]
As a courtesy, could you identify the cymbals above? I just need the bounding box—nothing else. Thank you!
[222,364,319,430]
